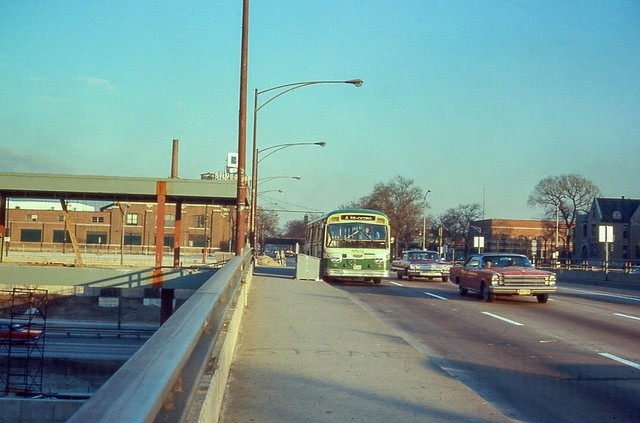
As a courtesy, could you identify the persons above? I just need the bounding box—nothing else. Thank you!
[363,227,371,240]
[509,259,517,265]
[483,258,495,268]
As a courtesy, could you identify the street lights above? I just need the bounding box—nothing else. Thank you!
[257,189,282,196]
[257,176,301,190]
[256,202,280,208]
[249,79,364,248]
[555,195,567,248]
[253,142,327,241]
[421,188,432,252]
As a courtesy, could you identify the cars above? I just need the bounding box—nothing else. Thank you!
[6,310,45,366]
[392,249,452,282]
[449,253,556,303]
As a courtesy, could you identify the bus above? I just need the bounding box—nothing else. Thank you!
[264,237,305,254]
[304,209,395,283]
